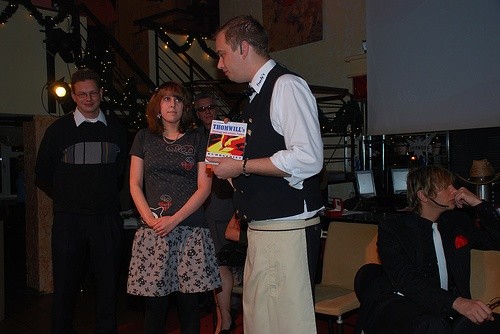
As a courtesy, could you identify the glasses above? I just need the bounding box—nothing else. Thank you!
[73,90,100,98]
[196,104,217,113]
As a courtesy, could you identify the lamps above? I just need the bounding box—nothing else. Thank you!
[46,77,77,114]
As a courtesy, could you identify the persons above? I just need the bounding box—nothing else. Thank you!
[127,81,222,334]
[194,90,248,334]
[354,165,500,334]
[33,68,130,334]
[207,15,324,334]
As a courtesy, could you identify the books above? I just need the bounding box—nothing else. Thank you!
[205,120,248,168]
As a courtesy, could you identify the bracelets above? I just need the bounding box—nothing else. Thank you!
[242,157,251,177]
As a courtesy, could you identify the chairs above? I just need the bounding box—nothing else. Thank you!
[314,222,381,334]
[451,248,500,334]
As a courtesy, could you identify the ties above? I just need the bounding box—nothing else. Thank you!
[432,222,449,291]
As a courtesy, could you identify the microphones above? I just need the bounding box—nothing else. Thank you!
[429,198,449,207]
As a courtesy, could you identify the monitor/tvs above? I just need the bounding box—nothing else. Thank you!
[392,169,410,194]
[355,170,376,198]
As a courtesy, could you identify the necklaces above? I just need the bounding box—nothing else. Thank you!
[162,131,179,145]
